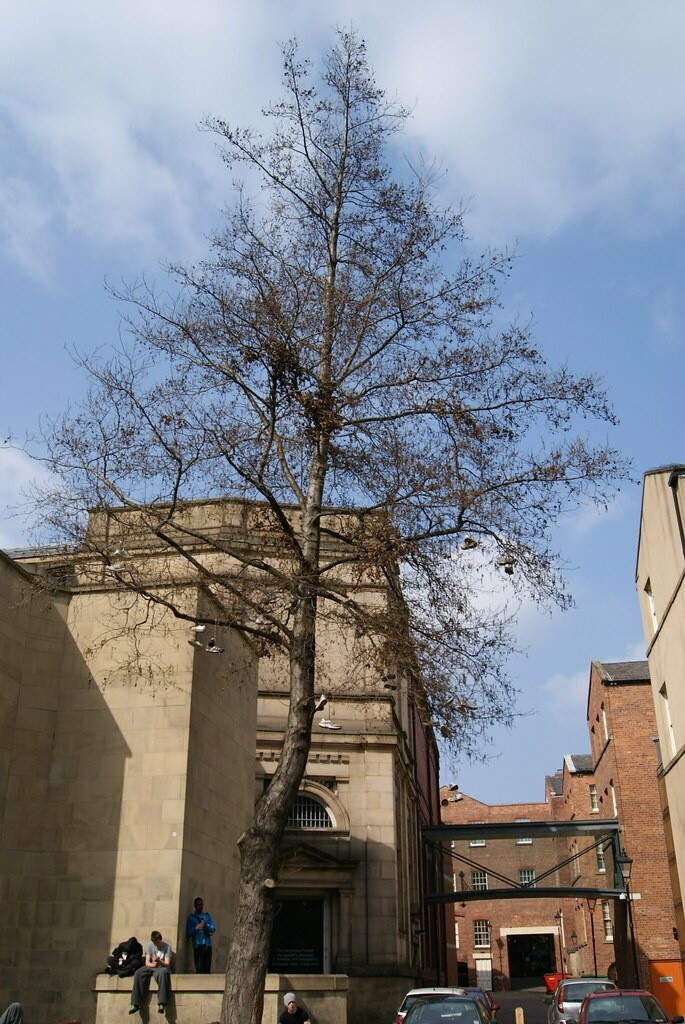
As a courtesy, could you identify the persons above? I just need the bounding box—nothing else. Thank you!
[0,1003,24,1024]
[186,897,216,974]
[280,992,312,1024]
[128,930,173,1015]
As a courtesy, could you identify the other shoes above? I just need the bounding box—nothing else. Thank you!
[158,1004,165,1013]
[129,1005,140,1014]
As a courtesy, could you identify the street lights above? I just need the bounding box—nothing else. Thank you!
[587,898,597,978]
[554,911,564,980]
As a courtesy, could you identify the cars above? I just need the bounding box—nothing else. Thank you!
[542,977,620,1024]
[398,987,468,1024]
[401,995,497,1024]
[460,987,500,1019]
[566,989,685,1024]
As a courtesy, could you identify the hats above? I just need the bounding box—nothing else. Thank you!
[283,992,297,1008]
